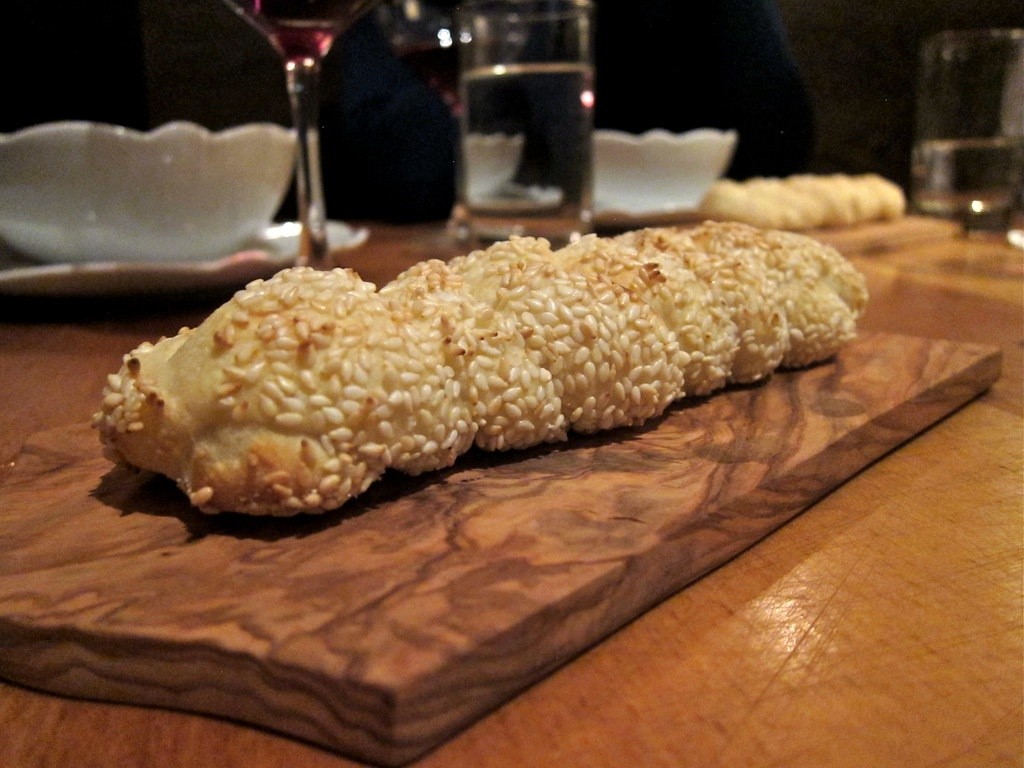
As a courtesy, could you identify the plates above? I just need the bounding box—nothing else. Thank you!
[1,217,369,305]
[796,216,962,270]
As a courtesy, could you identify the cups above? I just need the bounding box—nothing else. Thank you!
[910,29,1024,232]
[455,0,595,248]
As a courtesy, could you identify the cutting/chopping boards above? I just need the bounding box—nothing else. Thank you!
[0,328,1005,768]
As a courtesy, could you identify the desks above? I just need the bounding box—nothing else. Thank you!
[0,204,1024,768]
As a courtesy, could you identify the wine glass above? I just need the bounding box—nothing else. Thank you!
[224,0,383,274]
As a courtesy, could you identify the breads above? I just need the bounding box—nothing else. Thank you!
[89,215,870,515]
[700,172,907,231]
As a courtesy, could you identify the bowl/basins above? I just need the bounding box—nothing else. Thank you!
[0,121,320,265]
[463,126,742,231]
